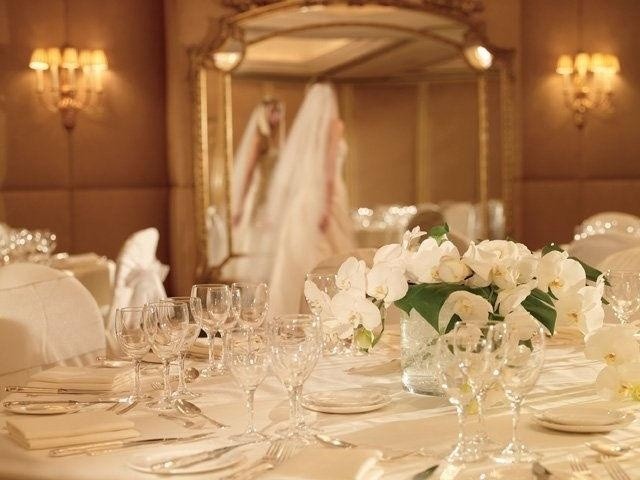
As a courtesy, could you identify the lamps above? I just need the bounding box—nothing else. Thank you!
[556,49,621,127]
[30,44,109,125]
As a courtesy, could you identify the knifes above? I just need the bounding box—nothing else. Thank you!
[2,384,118,408]
[48,435,241,475]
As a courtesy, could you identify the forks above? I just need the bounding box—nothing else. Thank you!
[220,440,298,479]
[566,453,632,480]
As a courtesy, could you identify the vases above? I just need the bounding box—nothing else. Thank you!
[397,295,475,398]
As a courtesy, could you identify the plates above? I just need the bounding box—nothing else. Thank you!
[298,388,394,413]
[534,404,637,434]
[6,405,69,414]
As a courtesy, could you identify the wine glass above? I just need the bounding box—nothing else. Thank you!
[430,315,546,462]
[115,270,358,447]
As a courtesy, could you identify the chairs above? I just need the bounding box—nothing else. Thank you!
[570,210,639,284]
[1,221,160,393]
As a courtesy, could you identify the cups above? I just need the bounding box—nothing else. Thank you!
[599,271,640,325]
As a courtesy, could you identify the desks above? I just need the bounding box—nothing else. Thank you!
[1,309,640,480]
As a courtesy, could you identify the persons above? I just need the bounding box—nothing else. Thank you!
[269,83,348,315]
[231,95,285,253]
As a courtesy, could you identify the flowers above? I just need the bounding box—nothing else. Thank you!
[322,229,608,367]
